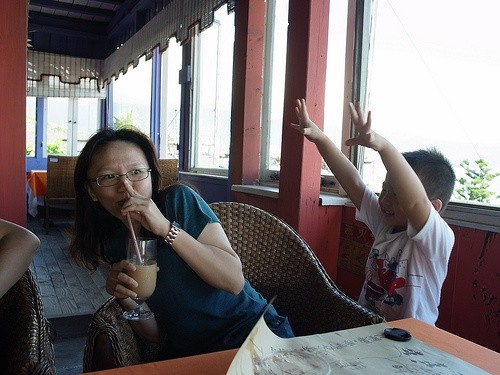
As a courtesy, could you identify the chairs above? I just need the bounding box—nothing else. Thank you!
[0,268,57,375]
[158,159,178,189]
[82,202,384,372]
[42,154,80,234]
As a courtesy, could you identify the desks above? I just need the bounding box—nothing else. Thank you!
[79,317,500,375]
[26,170,77,228]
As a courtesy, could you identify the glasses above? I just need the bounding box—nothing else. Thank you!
[87,168,152,187]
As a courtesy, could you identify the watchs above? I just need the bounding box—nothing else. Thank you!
[163,221,181,247]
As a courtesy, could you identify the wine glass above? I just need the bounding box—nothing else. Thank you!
[122,239,158,320]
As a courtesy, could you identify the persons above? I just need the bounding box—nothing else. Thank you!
[63,122,294,361]
[0,220,41,298]
[289,97,455,327]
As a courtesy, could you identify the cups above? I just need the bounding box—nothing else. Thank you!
[259,351,330,375]
